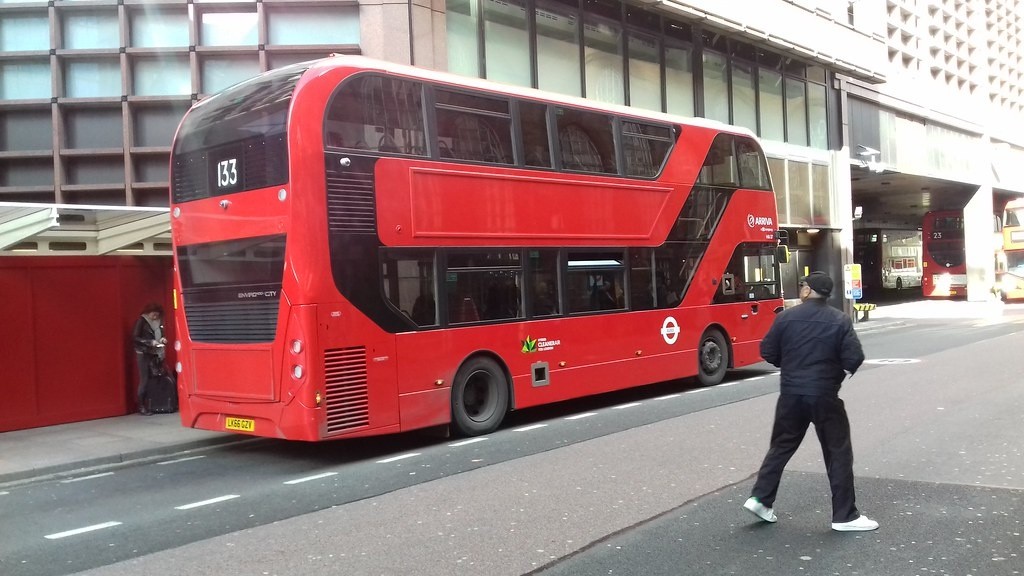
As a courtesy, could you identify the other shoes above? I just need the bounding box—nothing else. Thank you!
[140,406,151,416]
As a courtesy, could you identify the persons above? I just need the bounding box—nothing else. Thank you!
[598,281,616,309]
[637,271,675,311]
[744,271,879,530]
[485,276,522,320]
[133,302,168,416]
[532,279,558,316]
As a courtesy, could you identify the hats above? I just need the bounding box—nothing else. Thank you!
[801,271,832,297]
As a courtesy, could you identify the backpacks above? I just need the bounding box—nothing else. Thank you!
[145,376,176,412]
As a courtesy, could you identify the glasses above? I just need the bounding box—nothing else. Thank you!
[799,281,810,286]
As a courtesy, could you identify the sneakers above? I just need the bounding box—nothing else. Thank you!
[743,496,777,523]
[831,514,878,531]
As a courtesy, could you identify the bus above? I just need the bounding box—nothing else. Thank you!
[921,209,967,298]
[1001,196,1024,261]
[853,226,923,297]
[167,52,790,441]
